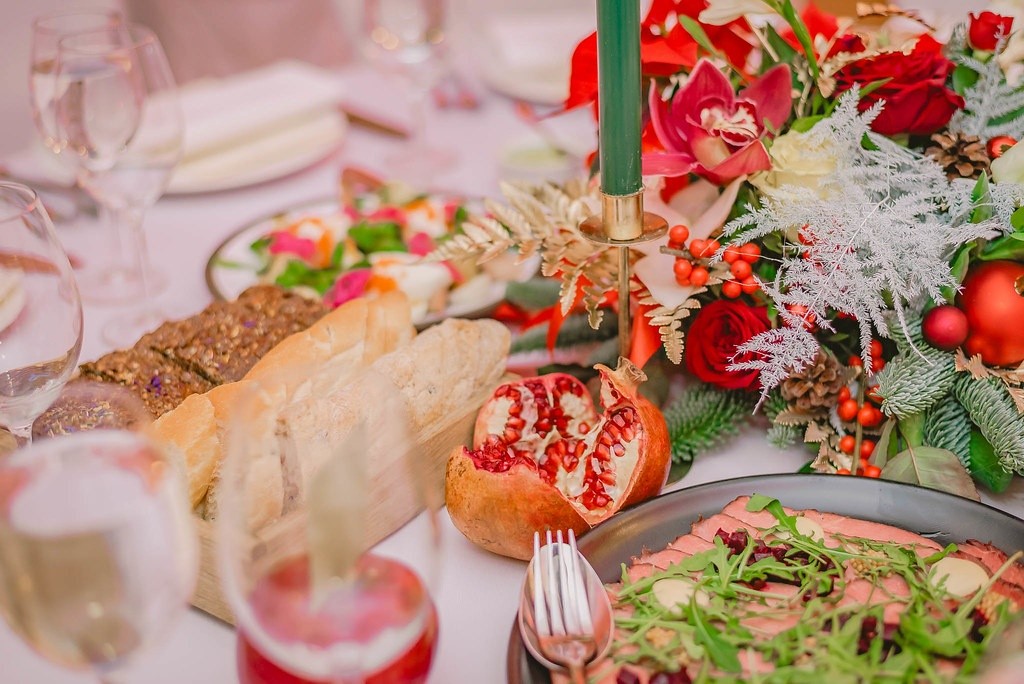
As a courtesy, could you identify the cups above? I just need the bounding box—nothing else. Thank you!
[1,433,199,684]
[216,369,440,684]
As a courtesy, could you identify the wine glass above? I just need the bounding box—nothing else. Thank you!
[328,0,458,182]
[0,181,84,451]
[30,13,167,303]
[56,27,187,345]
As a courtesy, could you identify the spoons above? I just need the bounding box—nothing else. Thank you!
[518,543,614,684]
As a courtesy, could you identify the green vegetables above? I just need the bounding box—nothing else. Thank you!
[583,494,1024,684]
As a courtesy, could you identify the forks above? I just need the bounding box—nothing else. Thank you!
[533,530,595,684]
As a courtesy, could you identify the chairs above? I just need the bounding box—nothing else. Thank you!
[120,0,360,100]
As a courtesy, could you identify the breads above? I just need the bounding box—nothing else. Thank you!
[0,280,513,546]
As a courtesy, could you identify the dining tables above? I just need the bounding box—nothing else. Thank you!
[0,0,1024,683]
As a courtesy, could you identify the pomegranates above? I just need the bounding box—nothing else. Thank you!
[445,357,670,561]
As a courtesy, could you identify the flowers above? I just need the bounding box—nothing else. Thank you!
[407,0,1024,511]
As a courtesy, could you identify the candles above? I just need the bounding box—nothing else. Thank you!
[595,0,644,241]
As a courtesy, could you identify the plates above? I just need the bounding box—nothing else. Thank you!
[205,200,600,367]
[2,101,346,194]
[505,476,1024,684]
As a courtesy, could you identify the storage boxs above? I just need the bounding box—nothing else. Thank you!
[188,371,522,627]
